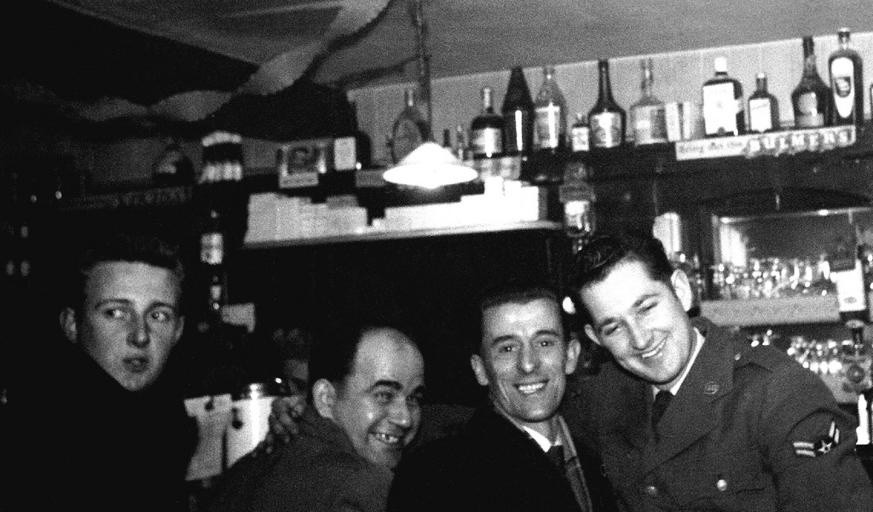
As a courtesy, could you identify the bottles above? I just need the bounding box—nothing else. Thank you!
[472,85,502,160]
[535,65,567,153]
[839,319,873,393]
[827,28,864,128]
[664,100,700,145]
[571,114,592,153]
[791,34,834,129]
[391,88,474,164]
[701,56,745,138]
[628,58,669,150]
[502,68,537,156]
[748,72,778,134]
[329,100,370,172]
[590,56,626,154]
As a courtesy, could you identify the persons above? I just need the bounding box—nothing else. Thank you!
[379,274,620,512]
[192,318,426,512]
[0,238,196,512]
[265,231,873,511]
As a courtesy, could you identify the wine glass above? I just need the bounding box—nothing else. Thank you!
[713,254,834,298]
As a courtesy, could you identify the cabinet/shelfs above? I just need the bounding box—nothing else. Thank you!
[0,125,873,465]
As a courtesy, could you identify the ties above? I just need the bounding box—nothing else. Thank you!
[548,445,566,478]
[651,389,675,432]
